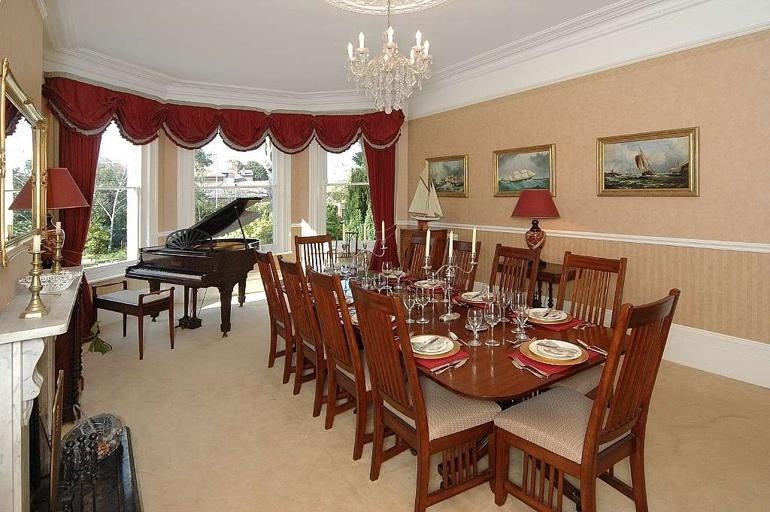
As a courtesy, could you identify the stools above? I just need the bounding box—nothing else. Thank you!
[92,279,175,360]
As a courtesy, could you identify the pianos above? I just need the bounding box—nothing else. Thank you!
[124,196,263,337]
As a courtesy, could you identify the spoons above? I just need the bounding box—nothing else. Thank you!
[449,332,470,348]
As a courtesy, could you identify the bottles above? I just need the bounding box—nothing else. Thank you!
[59,432,106,512]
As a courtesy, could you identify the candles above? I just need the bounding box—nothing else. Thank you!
[33,234,41,252]
[55,222,61,231]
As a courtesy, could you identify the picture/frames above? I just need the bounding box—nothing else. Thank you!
[595,126,701,198]
[0,56,48,269]
[424,153,469,198]
[492,143,557,198]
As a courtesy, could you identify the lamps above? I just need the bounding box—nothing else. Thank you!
[510,189,560,266]
[344,0,433,114]
[8,167,89,266]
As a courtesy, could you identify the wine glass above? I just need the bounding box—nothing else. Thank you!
[403,293,416,324]
[321,256,403,296]
[416,287,430,324]
[483,302,502,346]
[467,308,483,346]
[467,283,530,346]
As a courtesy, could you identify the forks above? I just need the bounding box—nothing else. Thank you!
[513,355,549,377]
[576,339,605,352]
[512,361,544,379]
[433,358,467,375]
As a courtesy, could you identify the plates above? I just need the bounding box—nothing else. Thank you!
[462,291,494,302]
[352,313,396,326]
[527,314,572,325]
[529,339,582,360]
[409,334,460,359]
[520,341,589,366]
[410,335,454,354]
[413,279,446,289]
[458,292,488,303]
[524,308,567,321]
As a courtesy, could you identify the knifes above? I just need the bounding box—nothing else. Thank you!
[578,343,607,356]
[429,359,462,372]
